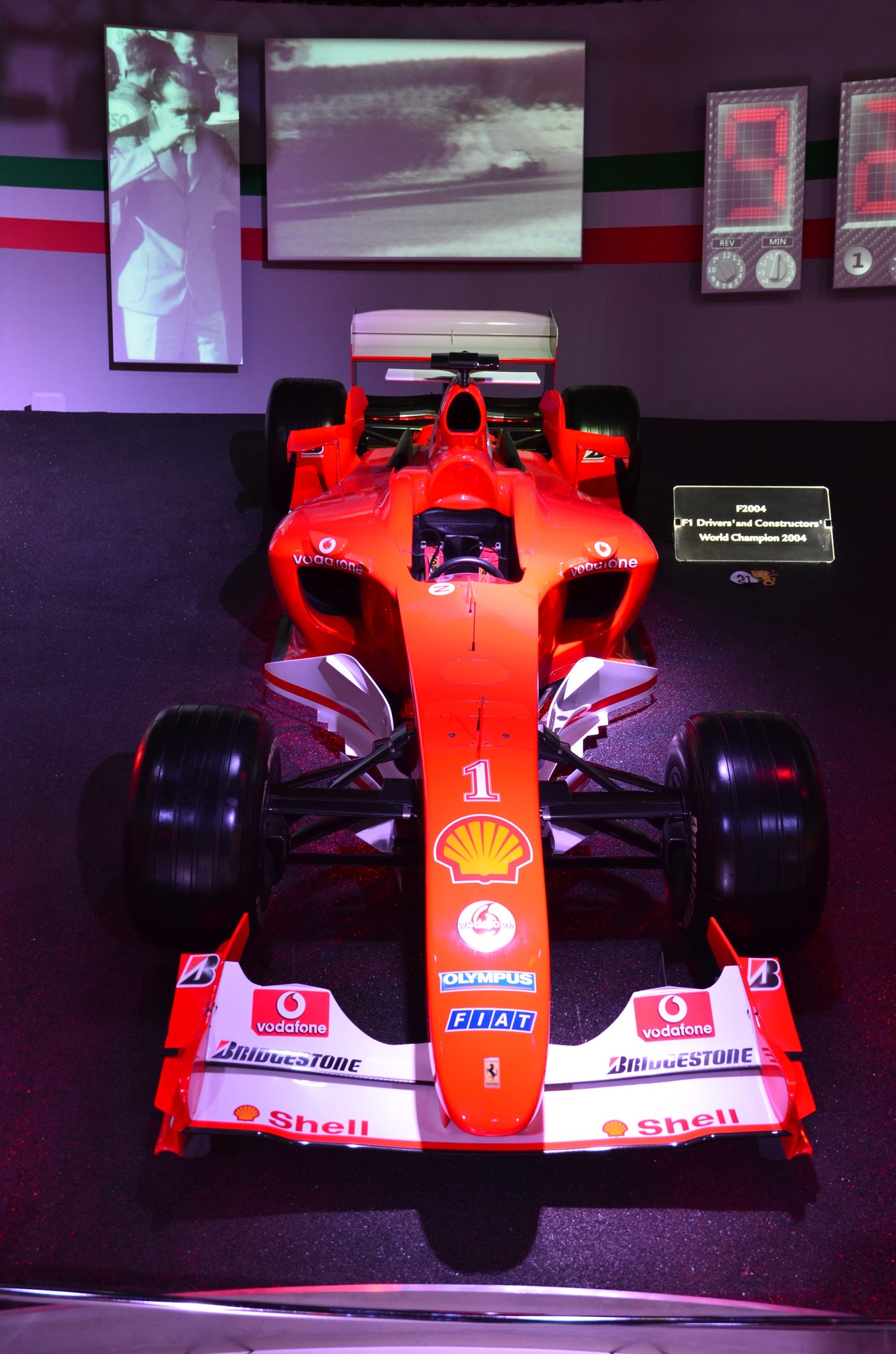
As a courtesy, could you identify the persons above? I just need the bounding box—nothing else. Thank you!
[105,30,239,365]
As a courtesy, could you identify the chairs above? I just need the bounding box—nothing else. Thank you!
[411,506,522,582]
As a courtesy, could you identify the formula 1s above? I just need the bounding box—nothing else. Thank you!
[123,301,830,1164]
